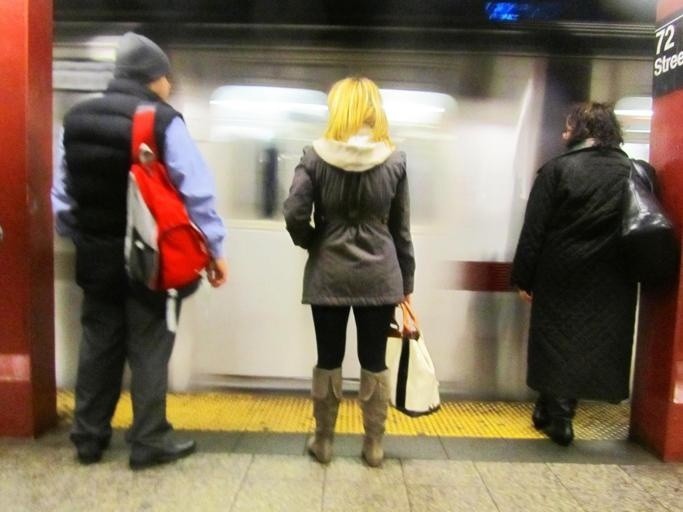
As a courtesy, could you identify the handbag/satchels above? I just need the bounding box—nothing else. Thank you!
[384,327,441,417]
[624,178,678,288]
[122,101,212,293]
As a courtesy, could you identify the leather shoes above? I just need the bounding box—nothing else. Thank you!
[129,439,197,469]
[78,442,104,464]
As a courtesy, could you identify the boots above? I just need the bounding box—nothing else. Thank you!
[359,369,391,467]
[532,396,575,446]
[307,365,343,463]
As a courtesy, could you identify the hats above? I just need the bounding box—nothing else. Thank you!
[114,30,172,85]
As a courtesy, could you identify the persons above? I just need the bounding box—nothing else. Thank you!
[509,98,657,446]
[281,75,416,466]
[48,29,228,470]
